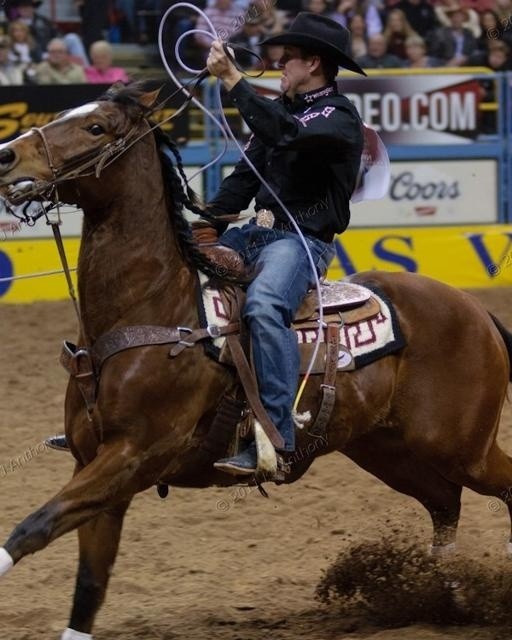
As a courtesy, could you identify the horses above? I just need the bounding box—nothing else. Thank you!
[0,77,512,640]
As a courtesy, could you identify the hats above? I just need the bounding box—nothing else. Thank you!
[255,10,368,78]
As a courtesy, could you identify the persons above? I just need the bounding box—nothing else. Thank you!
[44,9,366,482]
[0,0,510,82]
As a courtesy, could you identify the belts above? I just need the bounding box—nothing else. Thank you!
[255,208,332,245]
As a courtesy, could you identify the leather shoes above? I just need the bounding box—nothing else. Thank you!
[212,439,293,487]
[44,435,71,451]
